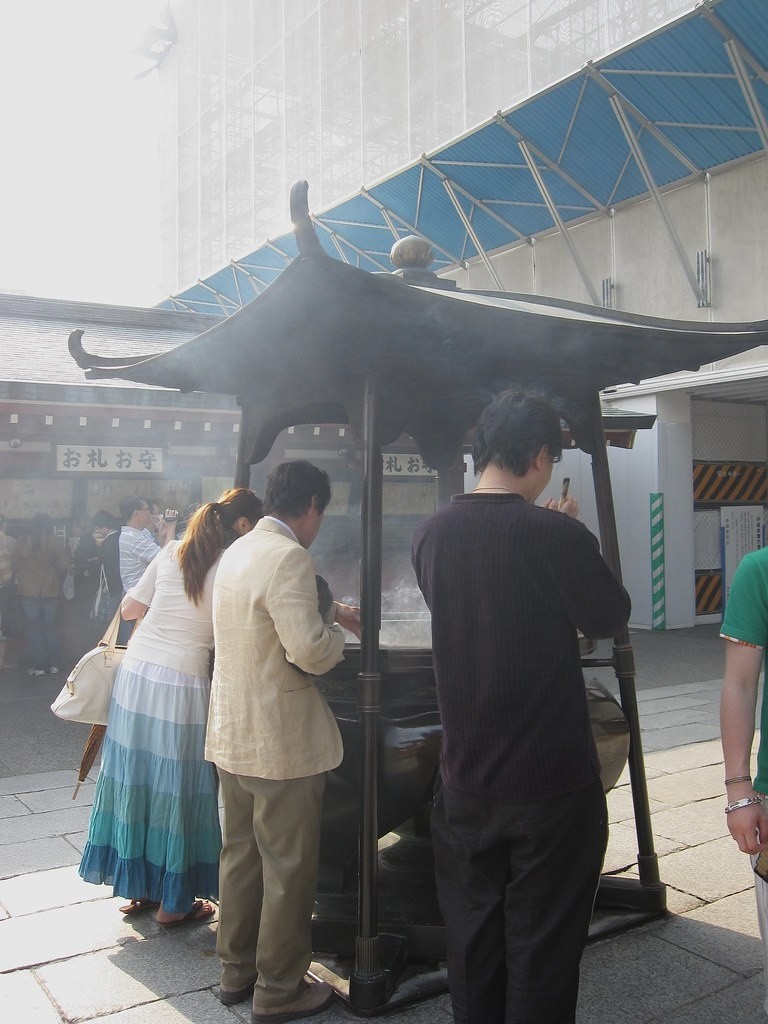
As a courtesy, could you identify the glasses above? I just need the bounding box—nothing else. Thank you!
[135,508,152,512]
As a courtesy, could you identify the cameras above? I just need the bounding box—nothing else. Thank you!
[161,512,179,520]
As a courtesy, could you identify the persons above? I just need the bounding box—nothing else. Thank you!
[79,488,264,928]
[410,389,632,1024]
[0,498,202,676]
[205,460,362,1024]
[720,549,768,1024]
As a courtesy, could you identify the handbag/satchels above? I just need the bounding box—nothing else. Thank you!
[51,603,144,725]
[90,562,119,621]
[63,573,75,601]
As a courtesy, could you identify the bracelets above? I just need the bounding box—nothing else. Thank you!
[724,796,762,814]
[724,775,752,785]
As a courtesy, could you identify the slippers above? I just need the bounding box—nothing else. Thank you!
[157,899,215,927]
[119,899,160,914]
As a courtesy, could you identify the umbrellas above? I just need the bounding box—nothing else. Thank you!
[72,612,145,800]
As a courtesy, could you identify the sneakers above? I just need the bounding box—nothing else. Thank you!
[219,982,255,1004]
[251,982,335,1024]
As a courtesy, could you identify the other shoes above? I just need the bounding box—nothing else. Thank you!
[50,666,59,673]
[0,662,16,669]
[28,666,46,675]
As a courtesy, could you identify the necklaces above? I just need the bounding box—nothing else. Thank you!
[472,487,512,493]
